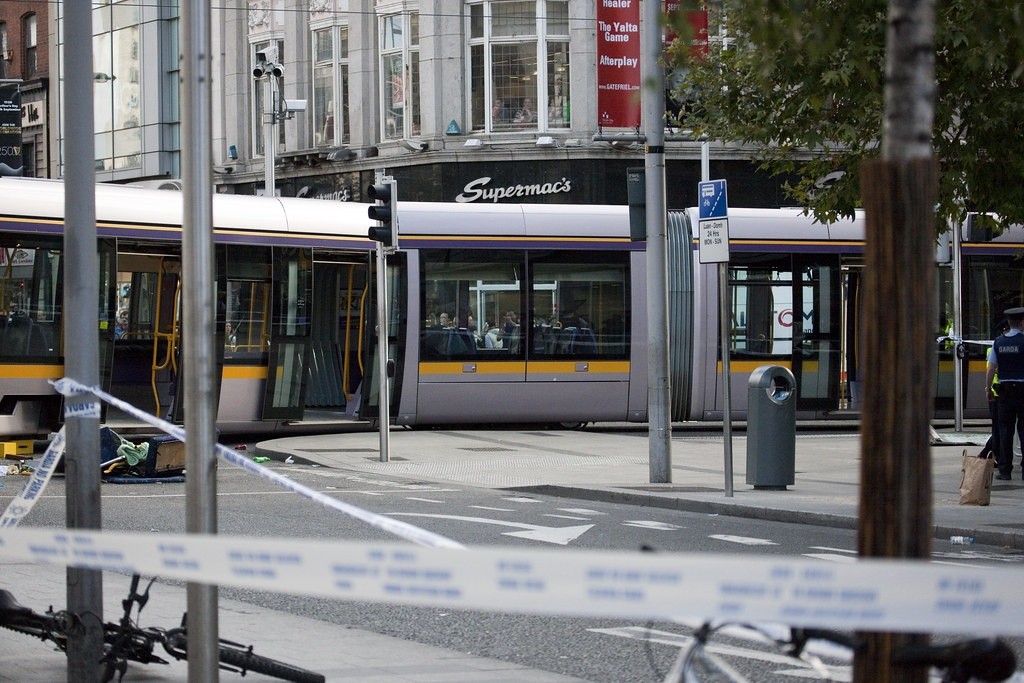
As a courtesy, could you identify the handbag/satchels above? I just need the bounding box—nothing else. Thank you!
[959,449,994,505]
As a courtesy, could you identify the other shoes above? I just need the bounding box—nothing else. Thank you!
[977,454,997,468]
[994,473,1011,480]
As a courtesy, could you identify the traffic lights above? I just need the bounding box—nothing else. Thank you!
[366,176,399,250]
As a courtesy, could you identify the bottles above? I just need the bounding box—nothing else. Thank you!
[950,536,974,545]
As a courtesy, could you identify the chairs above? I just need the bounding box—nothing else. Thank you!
[445,327,601,354]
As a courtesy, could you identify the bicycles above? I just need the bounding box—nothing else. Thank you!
[0,573,325,683]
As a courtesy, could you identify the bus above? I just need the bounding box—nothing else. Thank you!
[1,178,1024,453]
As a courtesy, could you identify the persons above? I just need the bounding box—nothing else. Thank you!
[492,96,563,124]
[976,307,1024,481]
[438,306,581,350]
[225,322,237,346]
[113,294,130,339]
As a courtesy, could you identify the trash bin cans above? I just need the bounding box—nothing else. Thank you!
[745,365,797,491]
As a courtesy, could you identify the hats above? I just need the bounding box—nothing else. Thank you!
[996,320,1010,332]
[1004,307,1024,320]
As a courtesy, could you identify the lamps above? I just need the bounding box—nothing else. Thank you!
[612,133,635,146]
[535,137,558,147]
[464,139,484,150]
[326,149,357,162]
[396,138,429,153]
